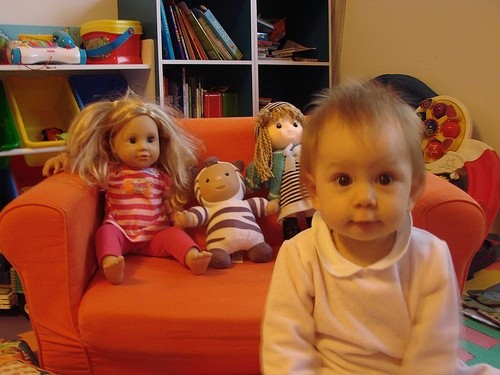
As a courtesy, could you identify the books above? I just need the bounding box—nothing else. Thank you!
[257,18,318,64]
[160,0,243,61]
[164,67,237,118]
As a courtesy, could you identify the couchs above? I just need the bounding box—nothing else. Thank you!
[0,115,486,375]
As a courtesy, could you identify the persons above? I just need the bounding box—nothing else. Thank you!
[260,78,461,375]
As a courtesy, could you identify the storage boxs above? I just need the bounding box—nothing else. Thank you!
[5,74,81,167]
[68,73,129,111]
[0,78,21,151]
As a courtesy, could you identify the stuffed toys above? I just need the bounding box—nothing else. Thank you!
[42,90,213,284]
[243,101,317,241]
[173,158,279,268]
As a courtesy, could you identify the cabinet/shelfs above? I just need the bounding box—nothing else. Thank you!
[0,39,156,208]
[117,0,332,117]
